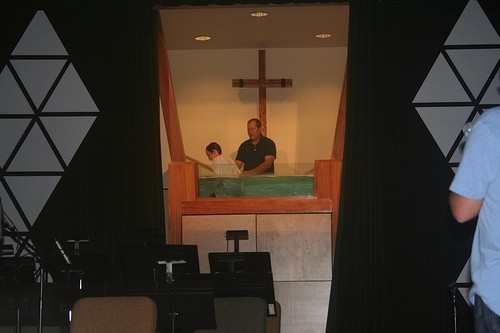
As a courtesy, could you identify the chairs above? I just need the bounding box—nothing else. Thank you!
[192,298,270,333]
[70,296,158,333]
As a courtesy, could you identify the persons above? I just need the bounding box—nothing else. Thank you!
[205,142,241,177]
[235,118,276,178]
[447,107,500,333]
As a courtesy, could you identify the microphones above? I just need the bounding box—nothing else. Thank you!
[463,122,474,138]
[56,241,72,265]
